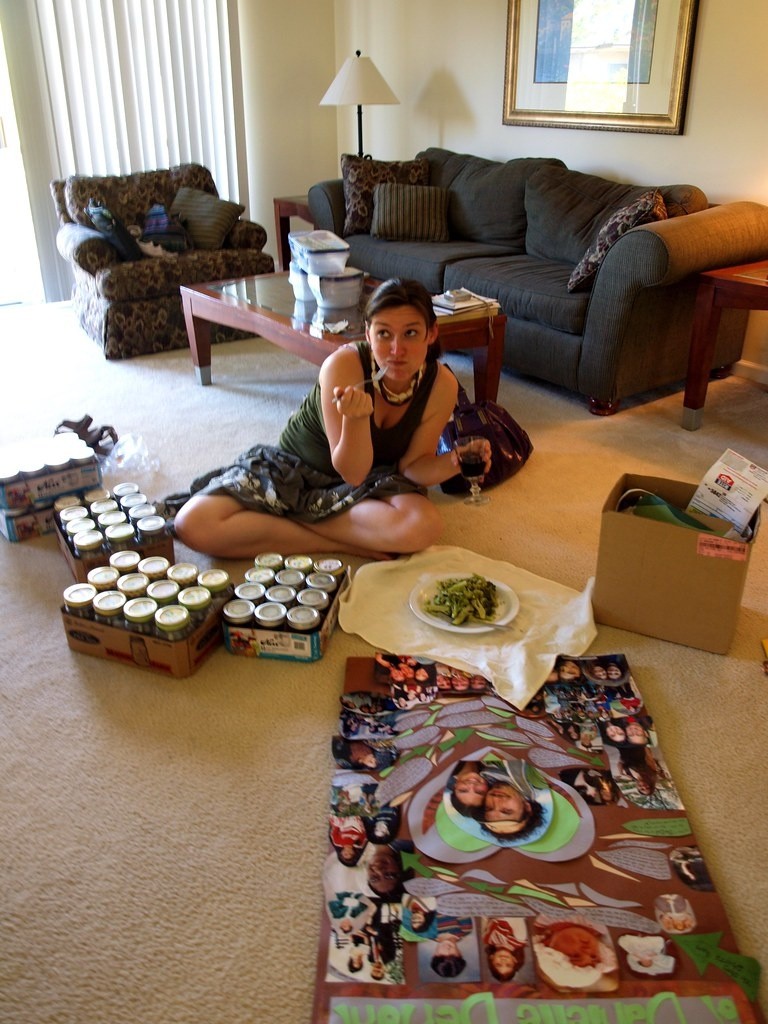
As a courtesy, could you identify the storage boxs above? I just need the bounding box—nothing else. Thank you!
[49,511,349,680]
[594,472,762,655]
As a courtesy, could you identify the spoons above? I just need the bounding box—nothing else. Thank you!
[331,365,389,405]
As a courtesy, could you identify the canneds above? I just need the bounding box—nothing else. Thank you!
[223,551,344,636]
[0,432,105,542]
[53,482,171,561]
[62,550,230,641]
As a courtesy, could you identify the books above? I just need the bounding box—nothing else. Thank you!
[432,287,502,324]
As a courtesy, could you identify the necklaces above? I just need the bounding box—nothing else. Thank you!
[371,351,423,407]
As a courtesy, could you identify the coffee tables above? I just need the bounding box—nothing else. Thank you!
[180,272,507,404]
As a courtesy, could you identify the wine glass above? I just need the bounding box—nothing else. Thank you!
[454,435,491,506]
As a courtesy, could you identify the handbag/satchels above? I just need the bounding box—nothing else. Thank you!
[83,197,144,262]
[140,202,193,251]
[436,363,535,496]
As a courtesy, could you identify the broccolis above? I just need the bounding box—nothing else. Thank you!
[423,572,499,625]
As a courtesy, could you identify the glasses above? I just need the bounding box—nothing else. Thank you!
[369,871,400,882]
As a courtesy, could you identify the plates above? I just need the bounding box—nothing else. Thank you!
[409,573,520,634]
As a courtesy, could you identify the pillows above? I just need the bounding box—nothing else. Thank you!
[341,153,431,238]
[171,186,245,249]
[567,186,669,293]
[85,197,142,260]
[371,182,448,242]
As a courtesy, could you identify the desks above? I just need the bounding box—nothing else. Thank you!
[273,195,320,271]
[682,259,768,432]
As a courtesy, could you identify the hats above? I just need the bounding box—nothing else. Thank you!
[478,816,530,834]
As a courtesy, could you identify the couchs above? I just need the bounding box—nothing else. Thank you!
[49,162,275,362]
[308,147,768,416]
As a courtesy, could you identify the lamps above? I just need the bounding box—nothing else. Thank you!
[318,50,400,160]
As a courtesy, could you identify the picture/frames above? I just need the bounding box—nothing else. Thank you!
[502,0,700,135]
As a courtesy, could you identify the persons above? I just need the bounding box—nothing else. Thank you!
[173,278,491,560]
[327,652,708,988]
